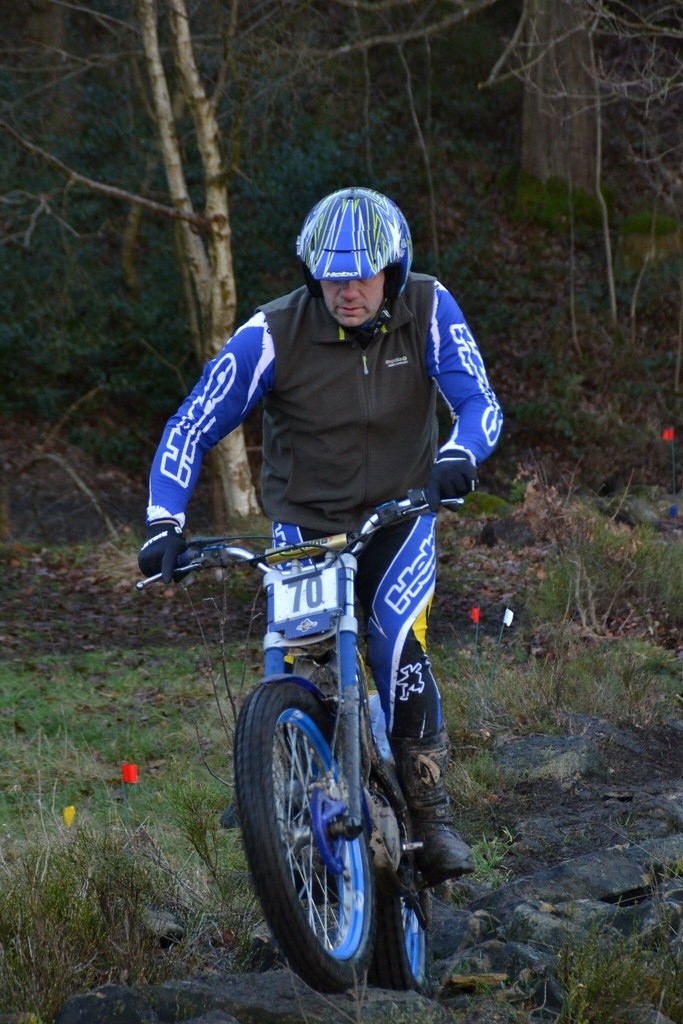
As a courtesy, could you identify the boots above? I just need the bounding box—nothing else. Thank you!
[218,753,239,829]
[387,727,476,872]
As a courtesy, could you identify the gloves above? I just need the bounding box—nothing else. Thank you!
[138,523,186,585]
[428,449,479,511]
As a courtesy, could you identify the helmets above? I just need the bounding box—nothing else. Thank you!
[297,187,413,300]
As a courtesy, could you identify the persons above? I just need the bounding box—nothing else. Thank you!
[136,184,505,881]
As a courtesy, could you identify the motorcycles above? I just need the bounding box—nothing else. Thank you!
[136,476,484,994]
